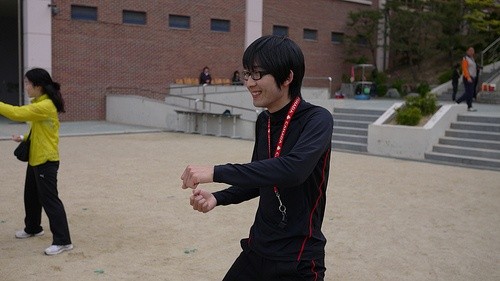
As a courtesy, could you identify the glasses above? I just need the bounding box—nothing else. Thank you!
[241,69,271,80]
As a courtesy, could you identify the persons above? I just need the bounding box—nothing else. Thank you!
[0,68,74,256]
[473,59,483,102]
[457,47,479,112]
[231,70,244,86]
[199,66,212,86]
[180,35,335,281]
[451,63,461,100]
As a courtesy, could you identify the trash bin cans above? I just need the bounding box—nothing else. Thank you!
[355,81,372,99]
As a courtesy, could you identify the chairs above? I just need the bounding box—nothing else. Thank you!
[175,78,232,86]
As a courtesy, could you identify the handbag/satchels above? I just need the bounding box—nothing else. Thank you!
[14,139,31,162]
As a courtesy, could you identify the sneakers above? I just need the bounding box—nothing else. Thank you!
[15,229,45,239]
[43,244,74,255]
